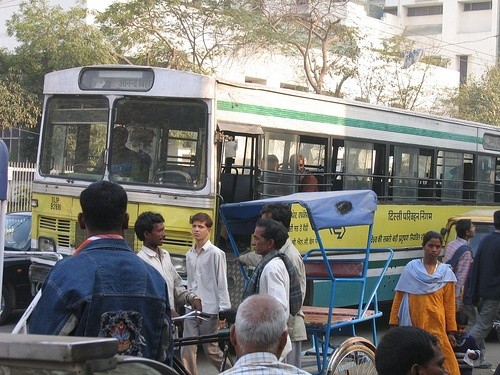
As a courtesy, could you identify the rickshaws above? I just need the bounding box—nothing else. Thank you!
[171,190,394,375]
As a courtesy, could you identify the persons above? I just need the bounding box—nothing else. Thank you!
[283,153,318,196]
[234,204,307,369]
[134,211,202,375]
[469,209,500,368]
[255,155,283,200]
[29,181,173,375]
[444,219,476,312]
[239,218,302,362]
[389,231,461,375]
[448,311,479,375]
[375,326,451,375]
[75,126,140,181]
[436,170,475,203]
[218,292,312,375]
[181,212,232,375]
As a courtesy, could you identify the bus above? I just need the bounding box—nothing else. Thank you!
[28,63,500,322]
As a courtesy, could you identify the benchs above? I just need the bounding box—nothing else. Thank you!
[289,260,375,328]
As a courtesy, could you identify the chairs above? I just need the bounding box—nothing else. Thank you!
[312,173,435,201]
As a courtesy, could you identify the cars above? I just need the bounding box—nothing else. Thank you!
[0,212,39,325]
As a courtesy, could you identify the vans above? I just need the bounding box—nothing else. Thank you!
[443,208,500,329]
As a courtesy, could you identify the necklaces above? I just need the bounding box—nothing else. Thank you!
[422,258,438,273]
